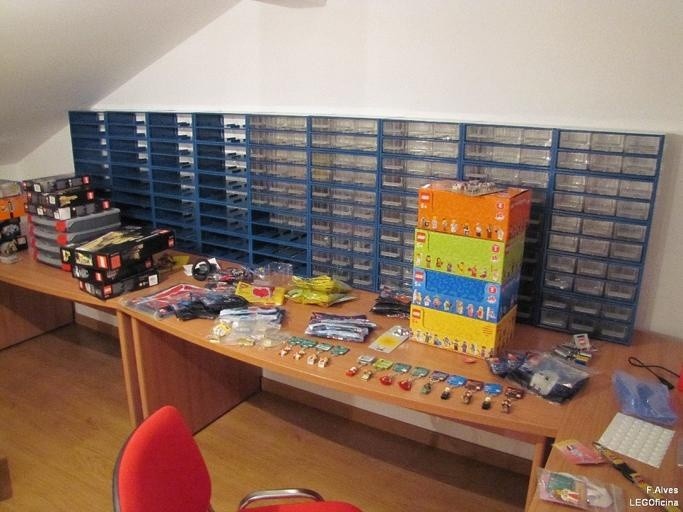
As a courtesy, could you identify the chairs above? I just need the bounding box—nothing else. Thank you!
[107,404,357,510]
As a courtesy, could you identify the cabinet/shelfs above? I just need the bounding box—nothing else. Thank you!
[307,113,380,293]
[461,124,555,328]
[67,109,246,263]
[537,127,664,347]
[247,110,307,280]
[375,119,460,302]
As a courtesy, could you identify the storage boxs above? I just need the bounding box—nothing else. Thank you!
[408,179,532,244]
[411,228,528,282]
[409,268,524,322]
[407,306,518,361]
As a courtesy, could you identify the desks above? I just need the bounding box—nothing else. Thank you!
[123,267,682,510]
[0,237,233,440]
